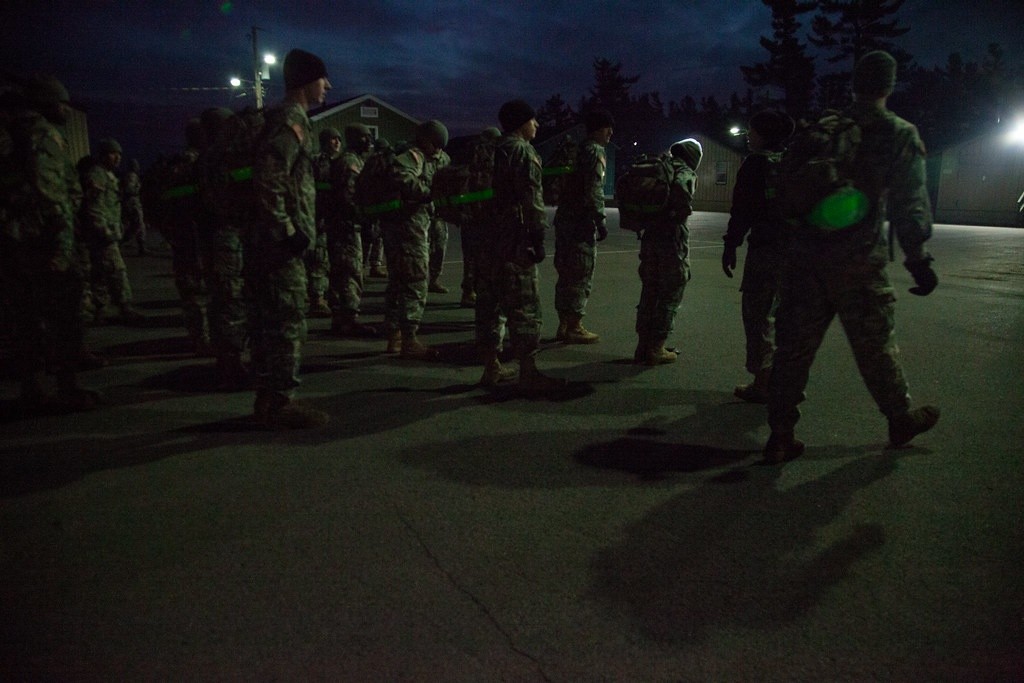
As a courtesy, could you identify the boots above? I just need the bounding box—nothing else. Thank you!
[385,320,440,360]
[251,381,331,426]
[481,351,568,398]
[308,295,333,317]
[765,421,806,464]
[889,405,938,446]
[369,263,388,278]
[634,333,678,366]
[428,274,450,293]
[90,302,148,325]
[556,317,598,344]
[734,371,776,404]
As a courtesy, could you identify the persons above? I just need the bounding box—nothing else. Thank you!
[0,48,567,432]
[553,111,614,343]
[721,110,806,404]
[634,137,704,366]
[759,50,940,465]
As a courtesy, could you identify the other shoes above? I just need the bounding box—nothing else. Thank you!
[459,280,477,308]
[328,305,376,337]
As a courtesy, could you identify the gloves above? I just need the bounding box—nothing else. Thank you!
[904,257,939,296]
[527,231,548,262]
[277,229,310,258]
[596,218,608,241]
[722,244,736,279]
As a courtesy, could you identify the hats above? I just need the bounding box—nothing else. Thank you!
[854,50,896,96]
[497,100,535,132]
[670,138,702,171]
[283,49,328,91]
[749,109,796,144]
[585,104,614,134]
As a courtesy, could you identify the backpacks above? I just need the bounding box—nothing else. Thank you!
[616,156,672,232]
[201,105,305,231]
[776,101,896,268]
[543,132,600,203]
[316,157,353,222]
[356,148,413,226]
[431,138,518,228]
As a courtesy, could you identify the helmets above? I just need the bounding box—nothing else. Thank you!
[414,119,449,148]
[34,75,72,104]
[100,137,123,154]
[181,106,267,138]
[480,127,502,141]
[320,122,410,154]
